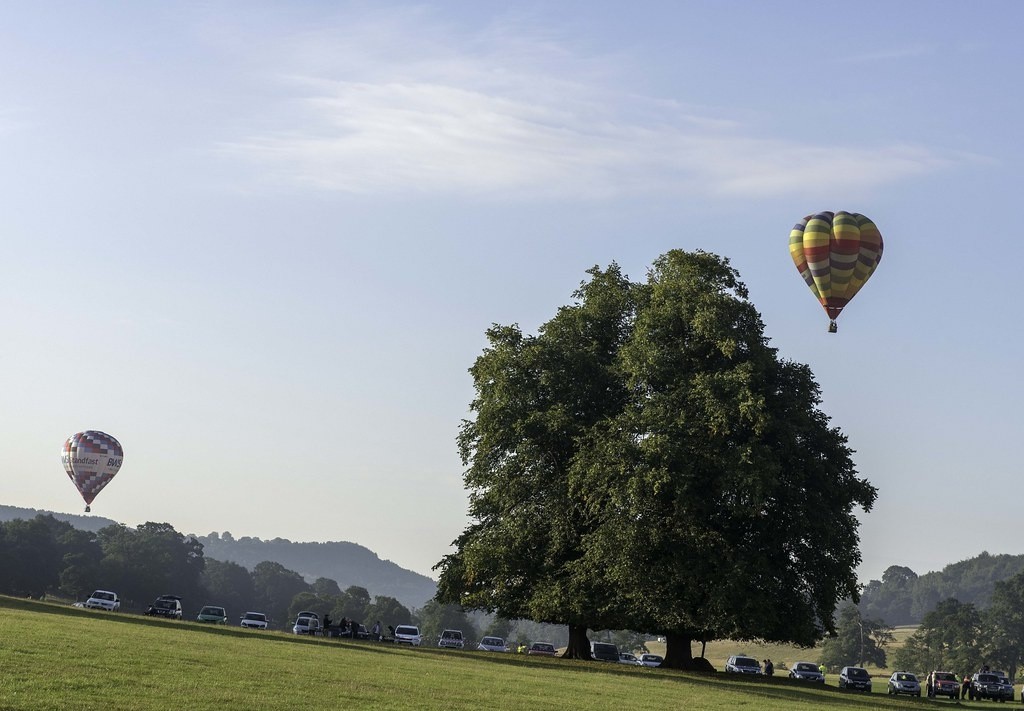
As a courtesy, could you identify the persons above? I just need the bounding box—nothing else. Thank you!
[925,671,934,698]
[763,659,775,678]
[323,614,333,638]
[516,642,527,656]
[387,624,396,636]
[308,614,317,636]
[371,620,381,641]
[960,675,972,700]
[340,616,359,639]
[819,663,828,679]
[954,672,961,683]
[27,593,33,600]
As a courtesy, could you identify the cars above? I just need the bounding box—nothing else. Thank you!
[692,656,718,673]
[239,611,270,630]
[291,611,322,636]
[196,606,229,626]
[638,652,663,667]
[619,652,642,666]
[85,590,120,612]
[788,661,826,684]
[476,635,509,653]
[337,623,371,640]
[839,666,873,693]
[887,670,921,699]
[527,641,559,658]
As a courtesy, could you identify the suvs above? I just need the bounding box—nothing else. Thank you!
[925,671,961,700]
[393,624,423,647]
[144,594,184,620]
[437,629,466,649]
[968,668,1015,703]
[724,654,762,674]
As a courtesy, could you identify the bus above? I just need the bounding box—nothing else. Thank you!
[590,641,622,664]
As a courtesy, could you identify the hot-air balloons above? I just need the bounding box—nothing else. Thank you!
[60,429,124,515]
[787,209,885,334]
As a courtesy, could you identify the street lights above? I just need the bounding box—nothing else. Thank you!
[858,623,864,668]
